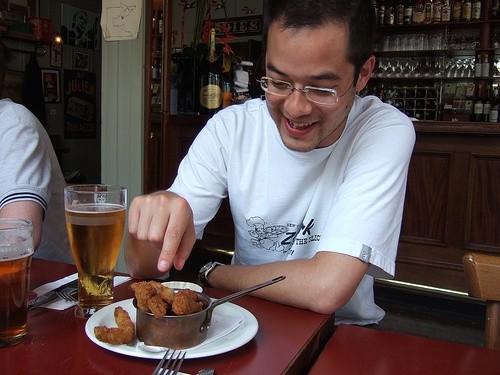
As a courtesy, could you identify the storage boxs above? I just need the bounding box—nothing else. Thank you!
[27,17,54,44]
[5,11,26,24]
[3,18,32,33]
[7,0,28,6]
[6,3,30,17]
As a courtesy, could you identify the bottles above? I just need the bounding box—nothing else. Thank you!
[471,84,483,122]
[199,27,223,114]
[159,13,163,35]
[474,54,482,78]
[152,59,159,82]
[372,0,482,25]
[483,85,500,122]
[386,90,434,121]
[482,55,490,78]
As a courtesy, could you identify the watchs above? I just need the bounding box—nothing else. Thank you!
[199,261,225,289]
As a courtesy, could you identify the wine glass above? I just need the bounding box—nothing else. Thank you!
[446,30,480,50]
[378,57,442,79]
[491,29,500,48]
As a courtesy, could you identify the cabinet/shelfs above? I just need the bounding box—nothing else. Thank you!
[367,0,500,123]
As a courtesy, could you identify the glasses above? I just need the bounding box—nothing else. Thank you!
[254,70,357,105]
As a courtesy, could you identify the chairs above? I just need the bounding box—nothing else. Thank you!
[462,251,500,349]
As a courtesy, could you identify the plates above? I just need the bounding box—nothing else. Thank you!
[85,297,260,358]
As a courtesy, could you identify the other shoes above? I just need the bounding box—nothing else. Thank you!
[64,170,80,180]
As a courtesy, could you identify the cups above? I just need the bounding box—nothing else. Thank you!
[0,217,34,348]
[64,183,128,316]
[381,34,443,52]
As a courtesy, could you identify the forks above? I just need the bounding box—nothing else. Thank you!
[152,348,186,375]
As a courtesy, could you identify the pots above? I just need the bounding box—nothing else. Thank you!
[133,275,286,350]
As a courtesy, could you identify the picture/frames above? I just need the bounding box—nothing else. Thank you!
[72,50,91,72]
[41,67,62,104]
[50,42,63,69]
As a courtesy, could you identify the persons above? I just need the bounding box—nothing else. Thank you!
[0,98,76,266]
[124,0,416,326]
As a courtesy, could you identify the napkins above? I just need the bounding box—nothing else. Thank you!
[31,269,131,312]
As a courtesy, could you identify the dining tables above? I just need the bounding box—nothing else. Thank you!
[305,323,500,375]
[0,258,334,375]
